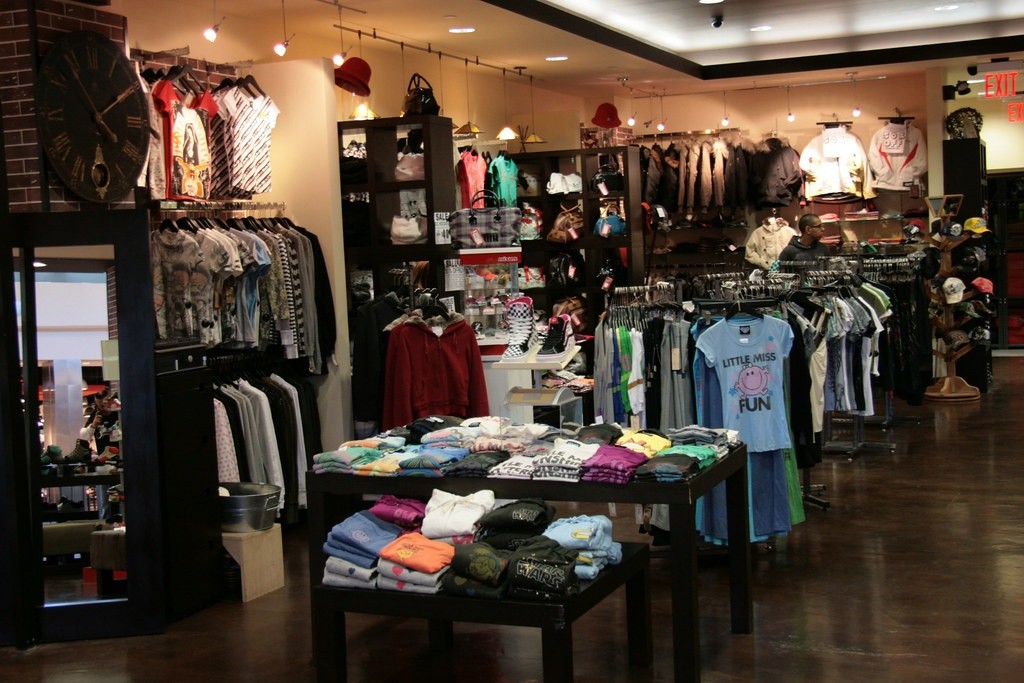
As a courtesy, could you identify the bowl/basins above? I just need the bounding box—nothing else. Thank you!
[219,482,282,534]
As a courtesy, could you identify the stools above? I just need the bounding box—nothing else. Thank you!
[222,524,285,603]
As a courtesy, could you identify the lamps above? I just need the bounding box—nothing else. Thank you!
[942,79,971,101]
[348,32,381,120]
[524,76,548,144]
[333,45,353,66]
[202,17,227,43]
[495,69,520,141]
[453,59,487,134]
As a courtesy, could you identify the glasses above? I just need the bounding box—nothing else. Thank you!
[810,223,824,228]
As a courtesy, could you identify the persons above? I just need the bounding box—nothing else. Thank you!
[78,381,119,520]
[778,213,830,273]
[743,216,797,271]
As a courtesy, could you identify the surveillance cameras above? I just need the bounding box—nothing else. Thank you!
[710,16,725,28]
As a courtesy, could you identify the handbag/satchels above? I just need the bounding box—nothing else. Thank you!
[516,265,546,290]
[590,164,624,196]
[553,295,589,333]
[592,204,626,239]
[394,152,425,181]
[516,170,539,196]
[447,189,522,249]
[390,215,428,245]
[546,201,584,242]
[400,72,441,116]
[545,249,585,291]
[545,172,582,194]
[600,254,624,291]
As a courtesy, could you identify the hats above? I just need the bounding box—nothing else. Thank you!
[650,205,672,226]
[641,202,652,234]
[334,57,372,97]
[964,217,992,235]
[591,103,622,129]
[927,220,994,350]
[910,219,928,231]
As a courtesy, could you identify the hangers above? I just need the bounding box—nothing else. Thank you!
[207,351,292,396]
[602,255,920,332]
[140,47,267,99]
[637,128,779,146]
[157,200,297,235]
[386,267,451,323]
[464,139,512,164]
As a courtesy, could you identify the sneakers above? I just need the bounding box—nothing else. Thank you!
[502,296,540,362]
[41,392,125,525]
[535,314,576,363]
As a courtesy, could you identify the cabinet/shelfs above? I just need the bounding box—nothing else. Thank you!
[41,473,121,522]
[510,146,644,334]
[942,137,994,394]
[458,246,523,363]
[337,115,456,342]
[821,216,930,246]
[644,224,748,258]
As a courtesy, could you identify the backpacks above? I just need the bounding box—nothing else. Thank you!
[518,202,543,240]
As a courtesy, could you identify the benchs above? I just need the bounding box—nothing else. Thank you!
[90,526,128,598]
[42,519,113,579]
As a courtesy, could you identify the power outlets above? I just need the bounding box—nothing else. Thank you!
[274,32,296,57]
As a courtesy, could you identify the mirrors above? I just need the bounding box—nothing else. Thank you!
[1,202,166,645]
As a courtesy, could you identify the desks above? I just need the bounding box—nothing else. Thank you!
[311,541,654,683]
[305,443,756,683]
